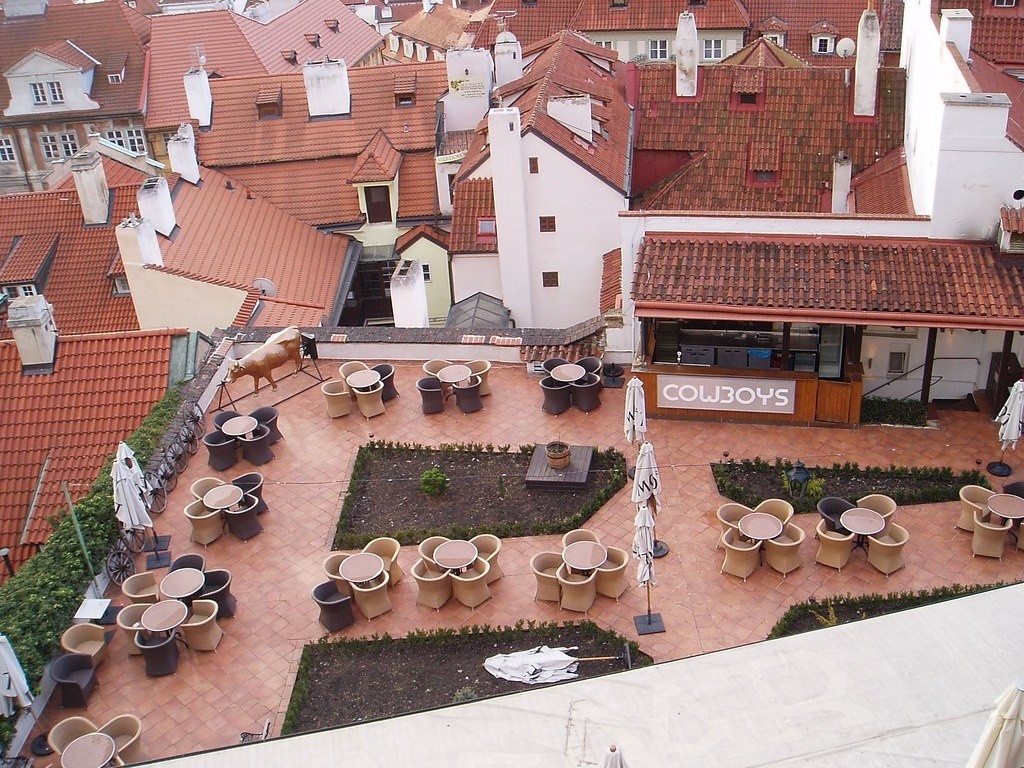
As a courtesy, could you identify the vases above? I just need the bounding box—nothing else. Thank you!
[545,442,571,469]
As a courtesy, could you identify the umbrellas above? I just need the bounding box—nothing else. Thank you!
[631,442,661,548]
[623,377,646,455]
[111,440,160,562]
[481,646,623,685]
[631,504,658,626]
[0,635,46,742]
[994,379,1024,465]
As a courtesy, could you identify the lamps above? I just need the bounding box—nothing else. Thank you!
[786,460,809,501]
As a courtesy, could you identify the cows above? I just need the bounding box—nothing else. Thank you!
[224,325,302,397]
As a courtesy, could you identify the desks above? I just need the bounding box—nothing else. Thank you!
[160,567,206,604]
[526,444,594,493]
[548,363,587,385]
[738,513,783,566]
[989,493,1024,542]
[436,364,471,400]
[561,541,608,576]
[72,598,123,626]
[141,601,189,652]
[346,369,381,391]
[62,733,115,767]
[202,486,244,531]
[431,540,478,576]
[841,509,886,556]
[223,416,258,437]
[338,553,384,585]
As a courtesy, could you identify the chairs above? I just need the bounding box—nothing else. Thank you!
[530,528,629,616]
[412,534,505,612]
[61,622,110,668]
[813,492,910,578]
[954,480,1024,560]
[239,719,271,745]
[185,472,270,549]
[415,359,493,414]
[321,360,399,421]
[311,536,405,632]
[538,357,604,414]
[716,497,805,583]
[52,653,99,709]
[47,714,144,768]
[204,406,284,472]
[118,553,238,675]
